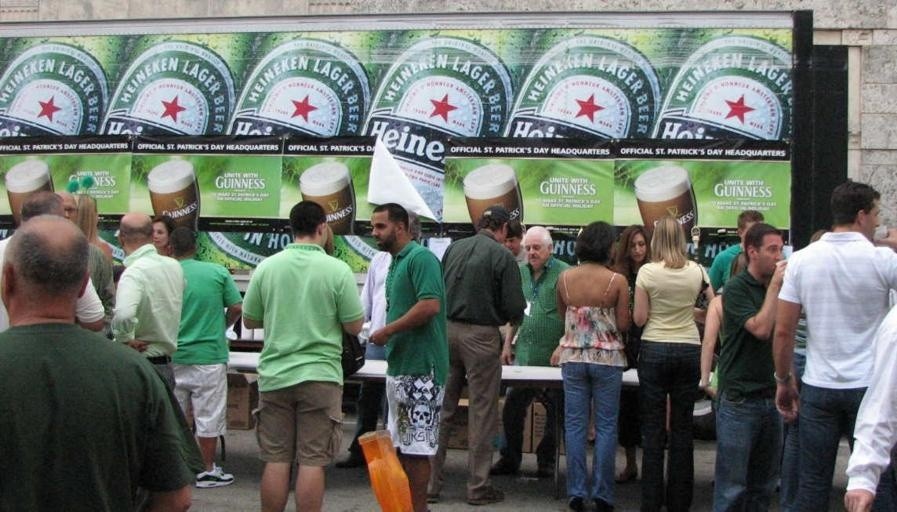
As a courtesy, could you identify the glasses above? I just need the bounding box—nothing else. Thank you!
[64,206,79,215]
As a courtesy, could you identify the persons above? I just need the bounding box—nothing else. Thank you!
[774,182,897,512]
[1,183,243,512]
[242,200,528,511]
[488,210,794,512]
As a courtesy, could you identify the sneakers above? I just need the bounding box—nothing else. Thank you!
[466,486,505,506]
[490,458,522,476]
[538,462,556,477]
[196,462,235,489]
[423,493,440,504]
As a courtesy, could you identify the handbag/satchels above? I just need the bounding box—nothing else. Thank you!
[341,333,366,379]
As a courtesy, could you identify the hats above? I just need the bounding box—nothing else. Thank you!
[480,205,511,220]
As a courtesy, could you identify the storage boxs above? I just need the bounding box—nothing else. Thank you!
[224,371,259,431]
[447,385,567,455]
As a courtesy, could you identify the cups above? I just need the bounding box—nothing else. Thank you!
[873,225,888,242]
[461,161,526,239]
[775,259,788,281]
[631,164,699,243]
[143,158,202,228]
[298,163,358,237]
[2,159,54,227]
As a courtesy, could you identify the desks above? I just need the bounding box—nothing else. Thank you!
[221,352,641,501]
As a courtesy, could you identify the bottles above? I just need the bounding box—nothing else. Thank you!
[359,28,533,141]
[227,31,391,141]
[359,428,415,512]
[653,28,793,143]
[500,27,682,142]
[0,37,129,138]
[99,35,253,138]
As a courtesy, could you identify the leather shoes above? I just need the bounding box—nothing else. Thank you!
[336,453,369,469]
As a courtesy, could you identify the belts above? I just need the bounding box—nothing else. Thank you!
[146,354,173,367]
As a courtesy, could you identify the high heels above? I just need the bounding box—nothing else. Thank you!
[568,493,584,511]
[590,495,615,512]
[615,465,638,483]
